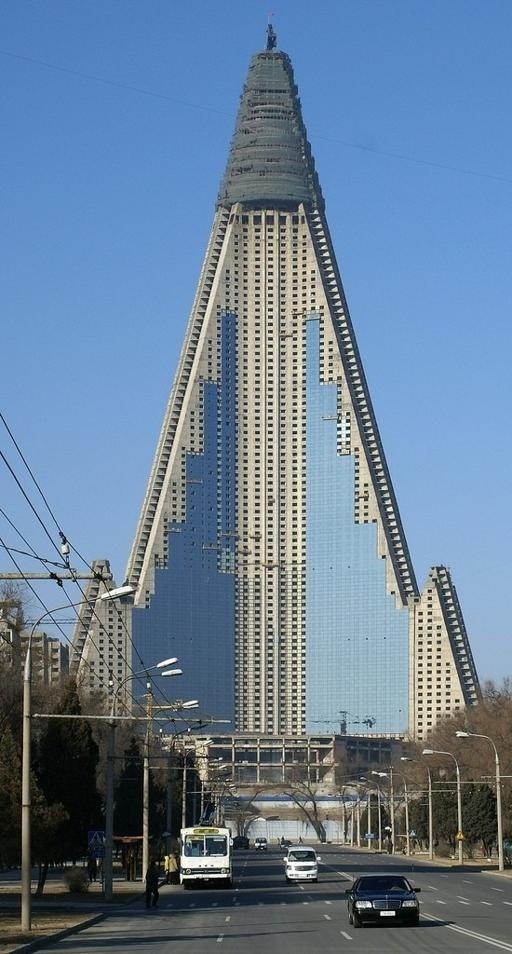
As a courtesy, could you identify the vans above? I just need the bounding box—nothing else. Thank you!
[284,846,321,883]
[254,837,268,850]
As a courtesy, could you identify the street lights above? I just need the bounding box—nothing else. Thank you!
[421,748,464,866]
[20,585,135,932]
[328,770,411,857]
[455,730,504,872]
[142,698,199,882]
[181,739,214,828]
[400,756,434,861]
[200,755,237,827]
[105,656,184,902]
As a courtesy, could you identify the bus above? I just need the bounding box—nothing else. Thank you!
[280,840,292,849]
[179,825,233,891]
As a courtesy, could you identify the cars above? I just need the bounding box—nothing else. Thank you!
[344,875,421,928]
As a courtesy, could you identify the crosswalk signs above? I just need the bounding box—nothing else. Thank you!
[409,829,416,837]
[89,832,104,846]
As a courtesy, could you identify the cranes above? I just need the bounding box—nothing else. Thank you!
[306,709,376,734]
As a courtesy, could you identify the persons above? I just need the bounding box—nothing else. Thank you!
[87,857,97,882]
[145,861,160,909]
[164,853,180,885]
[388,841,393,854]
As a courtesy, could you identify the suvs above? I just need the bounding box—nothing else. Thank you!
[233,836,250,849]
